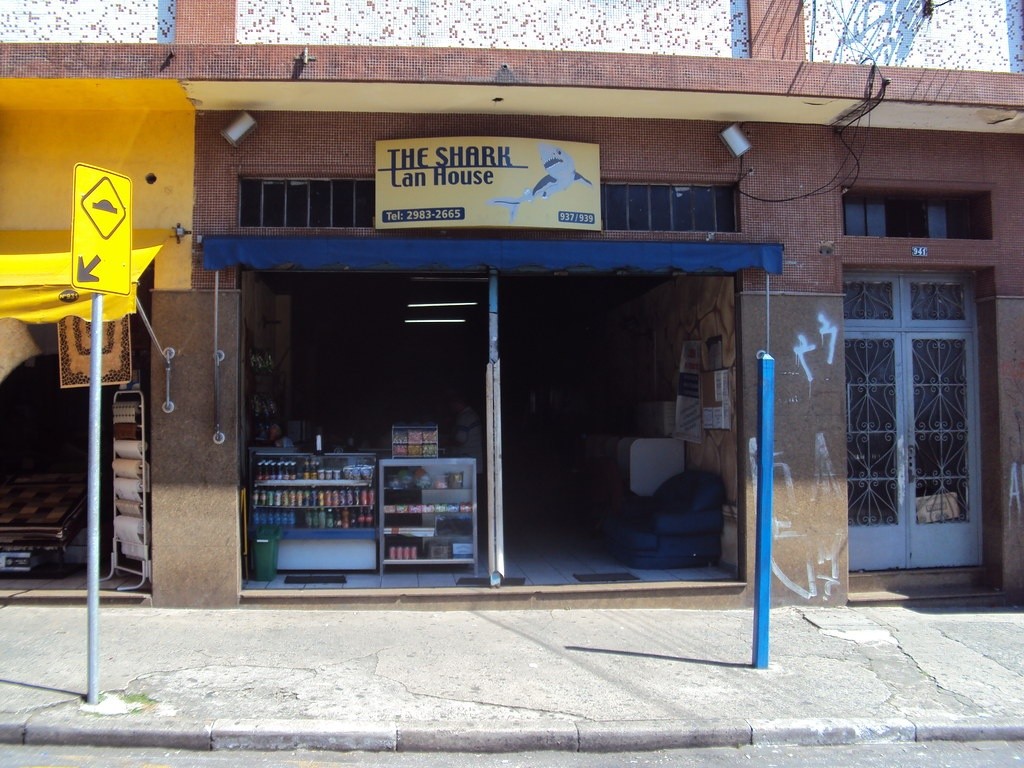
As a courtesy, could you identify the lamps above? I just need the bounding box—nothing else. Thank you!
[218,111,259,149]
[718,123,753,160]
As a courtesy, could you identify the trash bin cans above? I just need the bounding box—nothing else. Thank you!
[252,524,279,581]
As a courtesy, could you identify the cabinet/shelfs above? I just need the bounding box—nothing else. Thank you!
[99,390,153,591]
[247,445,377,570]
[379,458,478,578]
[392,422,438,458]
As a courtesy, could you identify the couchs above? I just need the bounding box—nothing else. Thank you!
[607,471,724,569]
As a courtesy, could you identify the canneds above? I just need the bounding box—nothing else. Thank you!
[389,546,417,560]
[251,487,376,506]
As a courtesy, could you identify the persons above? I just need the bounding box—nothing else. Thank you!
[450,390,485,524]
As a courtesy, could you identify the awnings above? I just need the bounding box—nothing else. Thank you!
[0,244,162,325]
